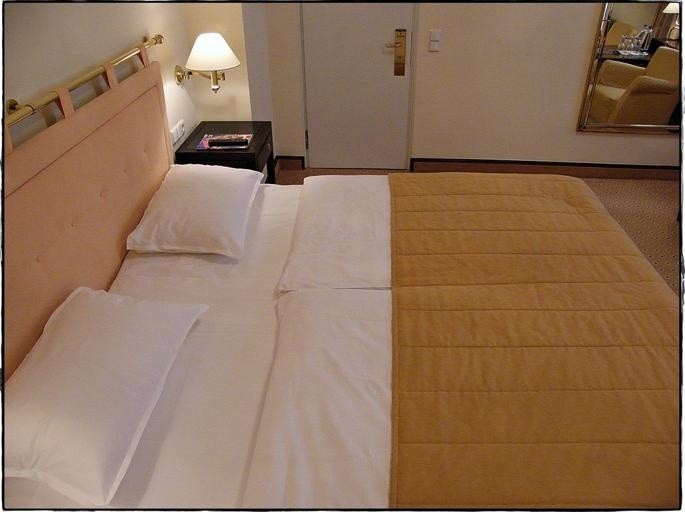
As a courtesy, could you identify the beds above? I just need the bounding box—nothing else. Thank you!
[1,36,682,509]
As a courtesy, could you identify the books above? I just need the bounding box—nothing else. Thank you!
[195,134,254,150]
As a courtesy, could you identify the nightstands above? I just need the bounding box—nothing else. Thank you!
[175,120,276,185]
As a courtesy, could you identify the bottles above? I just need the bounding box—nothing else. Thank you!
[637,23,654,53]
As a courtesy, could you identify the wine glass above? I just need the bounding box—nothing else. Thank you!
[619,35,643,52]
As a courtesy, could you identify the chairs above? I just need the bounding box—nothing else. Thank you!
[588,46,679,125]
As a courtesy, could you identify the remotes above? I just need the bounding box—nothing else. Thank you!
[208,139,248,146]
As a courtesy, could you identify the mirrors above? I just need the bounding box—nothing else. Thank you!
[578,3,680,133]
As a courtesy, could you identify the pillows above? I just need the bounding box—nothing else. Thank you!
[4,286,211,507]
[125,164,265,259]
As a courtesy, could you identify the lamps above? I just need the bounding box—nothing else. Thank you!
[661,2,680,15]
[174,33,243,94]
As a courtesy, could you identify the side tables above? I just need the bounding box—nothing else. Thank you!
[601,47,650,66]
